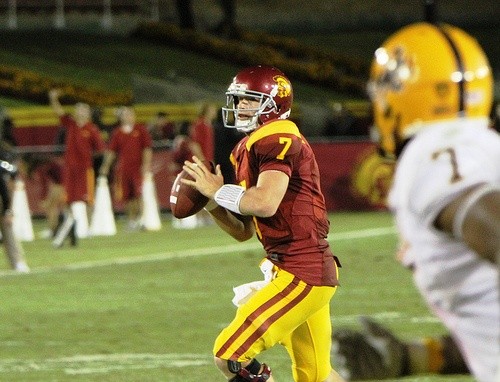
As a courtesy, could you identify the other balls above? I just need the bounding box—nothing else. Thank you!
[170,159,218,218]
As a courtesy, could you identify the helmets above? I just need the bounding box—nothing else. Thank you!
[222,65,293,132]
[372,21,494,162]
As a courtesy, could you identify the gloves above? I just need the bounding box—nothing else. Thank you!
[330,315,411,382]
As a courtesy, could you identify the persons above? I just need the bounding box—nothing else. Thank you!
[179,64,345,382]
[1,117,29,272]
[355,151,394,209]
[332,23,500,381]
[61,90,238,237]
